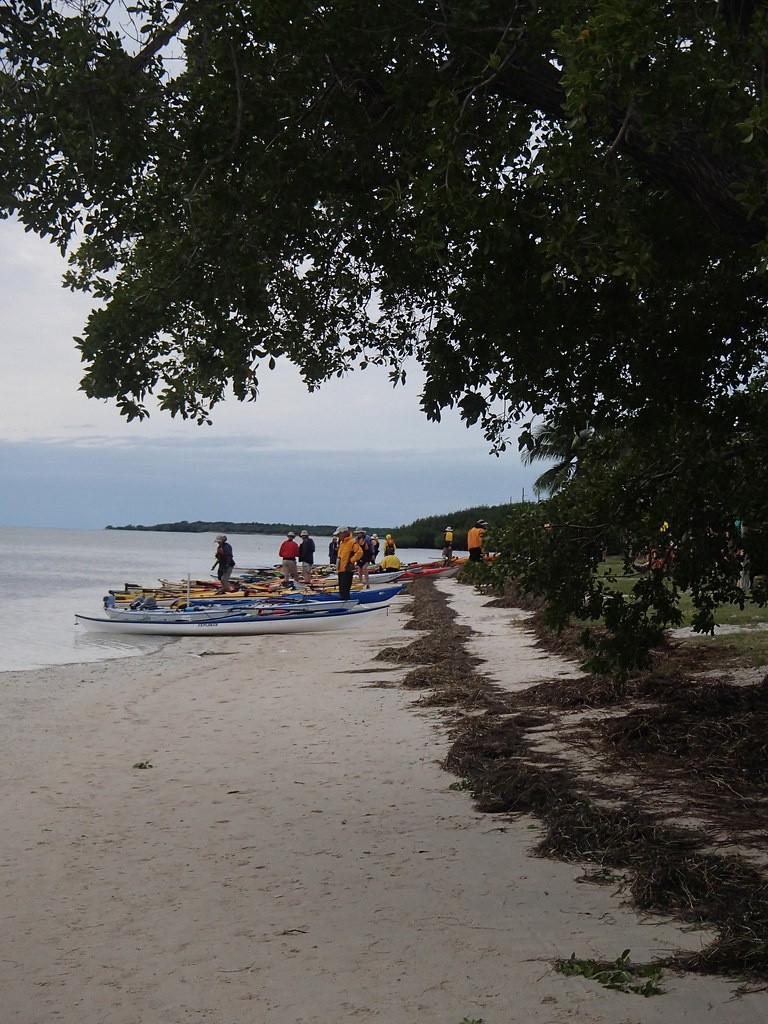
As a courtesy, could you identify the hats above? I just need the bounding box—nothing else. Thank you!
[287,532,295,538]
[445,527,453,531]
[477,520,488,526]
[371,534,378,539]
[300,530,308,536]
[333,536,337,540]
[333,525,348,535]
[354,527,366,533]
[214,535,222,542]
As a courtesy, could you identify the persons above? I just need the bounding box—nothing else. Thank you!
[354,526,376,588]
[279,532,299,583]
[380,549,401,572]
[738,561,751,588]
[371,534,380,565]
[333,526,364,600]
[467,519,488,561]
[215,535,236,594]
[384,534,396,557]
[329,537,338,565]
[635,550,678,570]
[443,527,454,568]
[298,529,315,583]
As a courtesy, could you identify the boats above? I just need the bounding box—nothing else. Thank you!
[73,552,501,634]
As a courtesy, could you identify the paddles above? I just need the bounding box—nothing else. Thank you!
[228,606,306,612]
[428,557,446,559]
[187,572,190,607]
